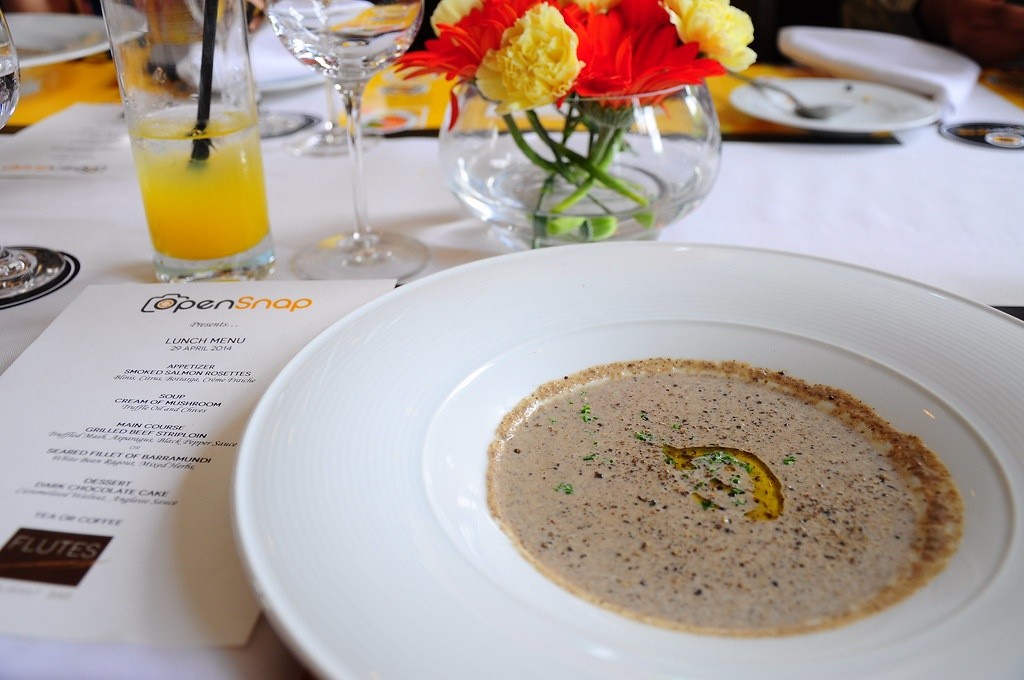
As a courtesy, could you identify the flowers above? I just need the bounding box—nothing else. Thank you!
[363,1,756,243]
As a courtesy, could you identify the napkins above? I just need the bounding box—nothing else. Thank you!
[184,0,375,96]
[778,28,987,127]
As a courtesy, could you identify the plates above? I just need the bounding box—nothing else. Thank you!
[176,0,383,92]
[0,0,148,71]
[728,77,942,134]
[231,242,1024,680]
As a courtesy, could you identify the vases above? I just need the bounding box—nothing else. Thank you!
[438,82,723,249]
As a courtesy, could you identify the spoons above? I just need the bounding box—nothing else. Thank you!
[726,71,855,120]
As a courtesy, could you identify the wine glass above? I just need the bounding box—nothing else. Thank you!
[0,0,66,299]
[264,0,430,281]
[183,0,308,138]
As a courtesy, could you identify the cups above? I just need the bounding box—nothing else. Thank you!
[99,0,277,284]
